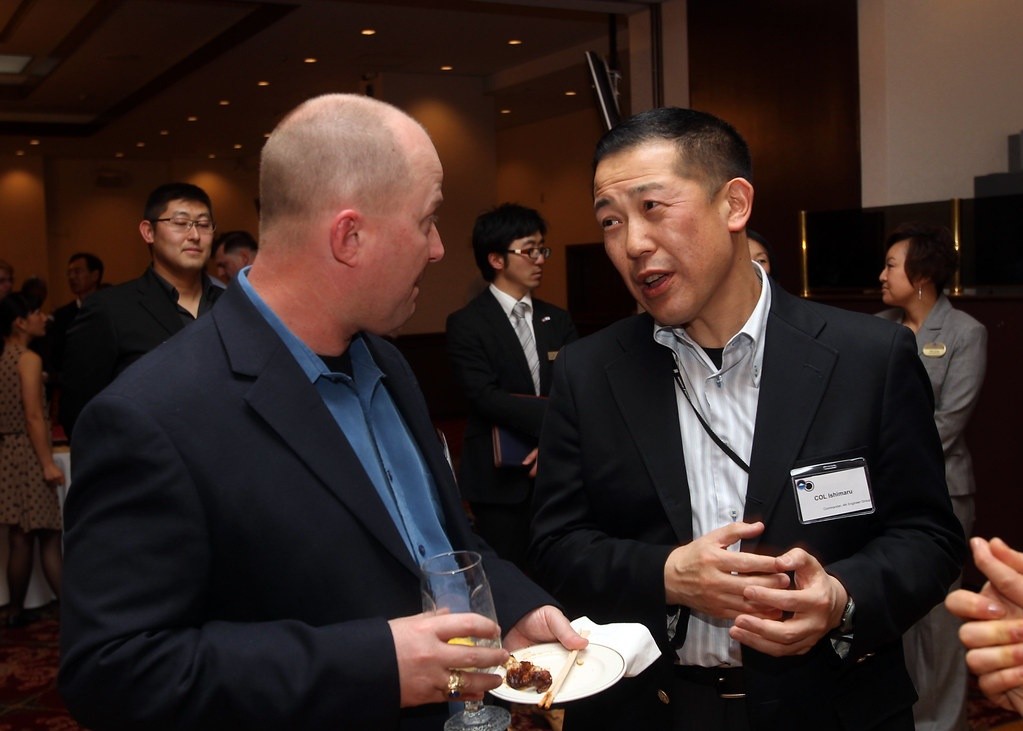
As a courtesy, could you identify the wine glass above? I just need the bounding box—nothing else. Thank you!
[420,550,512,731]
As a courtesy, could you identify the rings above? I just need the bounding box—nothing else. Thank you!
[445,670,463,699]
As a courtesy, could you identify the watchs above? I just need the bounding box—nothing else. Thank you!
[839,596,856,634]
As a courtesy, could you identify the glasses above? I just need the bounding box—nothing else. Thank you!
[157,218,217,235]
[508,247,550,260]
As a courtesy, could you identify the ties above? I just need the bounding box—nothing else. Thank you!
[512,303,540,396]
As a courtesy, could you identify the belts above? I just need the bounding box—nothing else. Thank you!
[675,668,748,699]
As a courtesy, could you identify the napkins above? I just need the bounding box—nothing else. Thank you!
[570,614,662,679]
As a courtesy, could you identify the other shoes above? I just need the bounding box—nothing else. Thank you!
[6,610,42,629]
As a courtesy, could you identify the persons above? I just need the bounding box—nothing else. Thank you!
[746,232,770,275]
[944,537,1023,717]
[214,230,258,282]
[517,107,965,731]
[872,219,989,731]
[59,92,588,731]
[0,182,224,628]
[445,204,580,568]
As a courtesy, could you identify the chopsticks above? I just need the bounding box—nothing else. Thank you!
[537,629,588,710]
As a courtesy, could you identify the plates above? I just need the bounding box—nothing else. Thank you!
[486,641,628,704]
